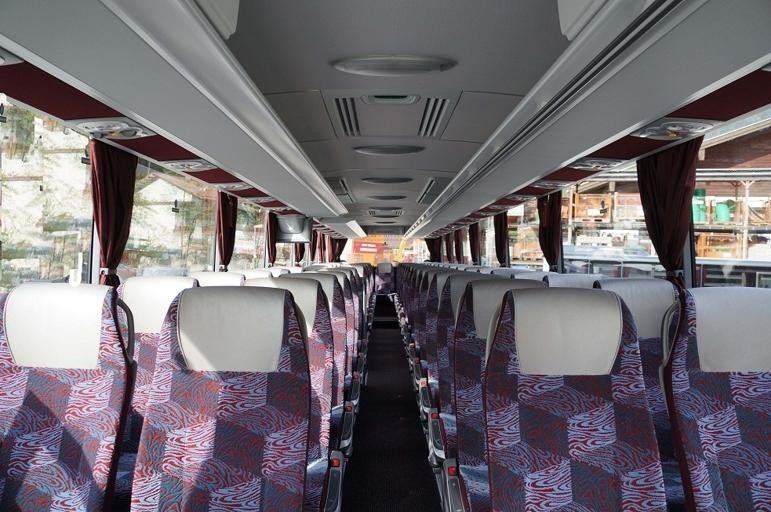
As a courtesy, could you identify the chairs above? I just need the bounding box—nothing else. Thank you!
[1,261,377,512]
[394,260,769,512]
[376,261,393,297]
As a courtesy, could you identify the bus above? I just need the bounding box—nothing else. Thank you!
[350,234,384,264]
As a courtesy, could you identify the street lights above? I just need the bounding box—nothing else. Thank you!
[252,222,263,269]
